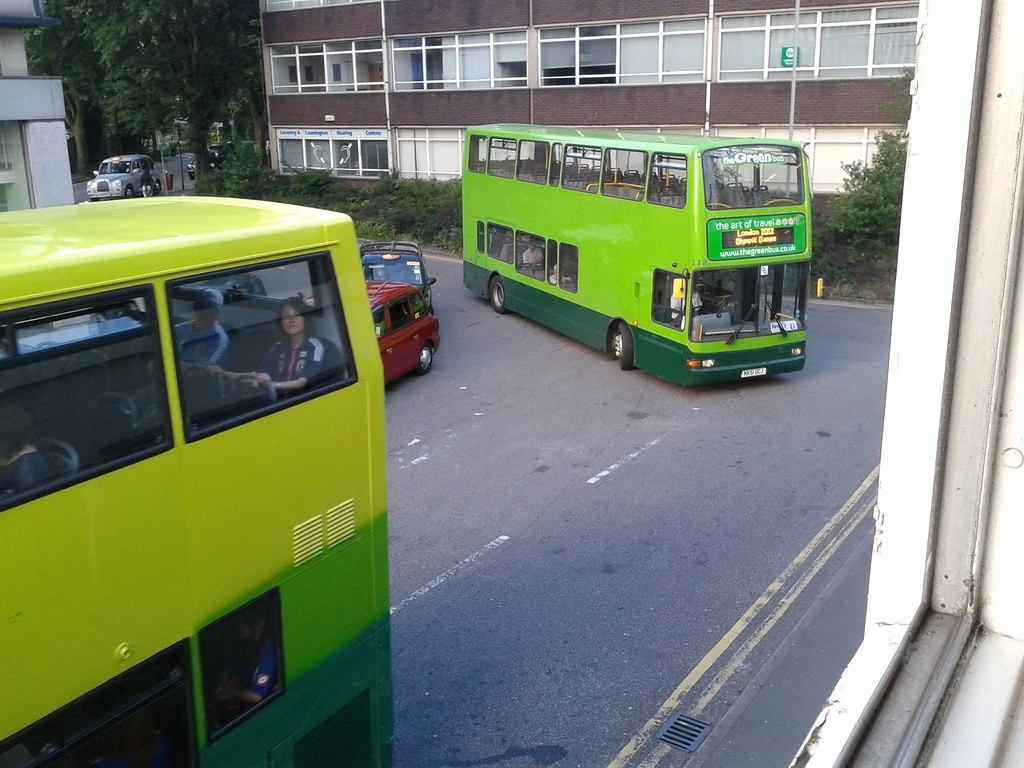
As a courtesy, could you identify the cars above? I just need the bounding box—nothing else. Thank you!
[87,155,162,201]
[209,143,223,154]
[187,150,218,180]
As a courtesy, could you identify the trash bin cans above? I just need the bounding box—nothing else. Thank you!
[166,173,173,191]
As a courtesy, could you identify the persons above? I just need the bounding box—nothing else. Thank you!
[145,287,226,382]
[548,263,573,288]
[668,286,702,319]
[521,243,544,266]
[206,297,327,396]
[219,609,278,701]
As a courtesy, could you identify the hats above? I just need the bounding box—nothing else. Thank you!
[196,288,224,310]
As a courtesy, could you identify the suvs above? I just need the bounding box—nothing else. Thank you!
[360,241,436,314]
[304,281,440,385]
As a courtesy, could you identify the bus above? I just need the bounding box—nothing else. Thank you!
[462,123,825,386]
[0,197,393,768]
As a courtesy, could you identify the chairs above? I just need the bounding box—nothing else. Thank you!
[0,309,349,490]
[565,278,577,292]
[468,156,772,211]
[530,262,545,282]
[518,262,532,275]
